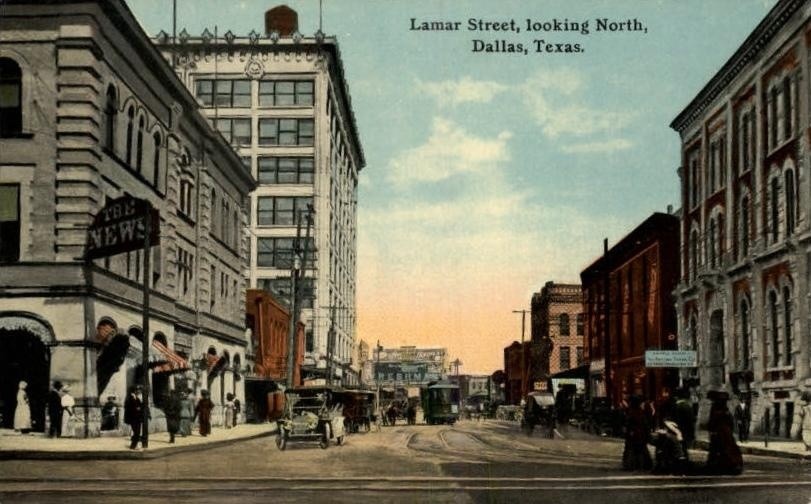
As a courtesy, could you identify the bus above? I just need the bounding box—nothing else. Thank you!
[421,381,461,426]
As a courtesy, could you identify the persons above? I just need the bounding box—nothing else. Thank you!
[193,388,215,436]
[60,382,77,438]
[551,388,751,477]
[178,391,195,435]
[164,388,182,443]
[48,380,61,438]
[14,379,35,434]
[223,392,237,428]
[102,394,120,429]
[123,384,144,450]
[136,382,152,447]
[233,393,241,426]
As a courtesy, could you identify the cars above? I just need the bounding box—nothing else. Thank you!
[464,396,489,419]
[276,377,416,451]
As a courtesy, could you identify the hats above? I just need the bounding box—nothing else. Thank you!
[53,381,63,389]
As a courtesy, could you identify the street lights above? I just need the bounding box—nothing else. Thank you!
[512,309,532,399]
